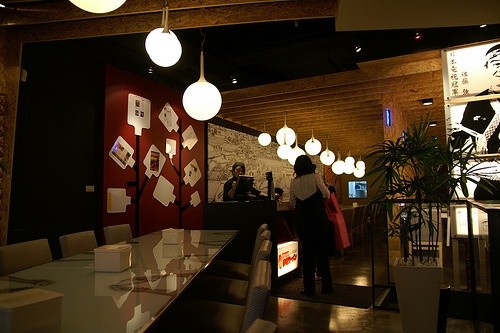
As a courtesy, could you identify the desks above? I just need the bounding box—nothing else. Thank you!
[0,230,238,333]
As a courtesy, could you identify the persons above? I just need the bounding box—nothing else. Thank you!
[289,155,336,296]
[451,44,500,200]
[223,162,261,201]
[275,188,284,201]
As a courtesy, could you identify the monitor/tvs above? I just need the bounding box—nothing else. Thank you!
[234,175,254,194]
[348,181,367,198]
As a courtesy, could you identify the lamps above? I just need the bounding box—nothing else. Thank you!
[145,0,222,121]
[257,114,365,178]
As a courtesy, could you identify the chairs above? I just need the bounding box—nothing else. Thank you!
[0,239,52,278]
[341,205,371,256]
[169,224,277,333]
[59,230,97,258]
[104,224,132,245]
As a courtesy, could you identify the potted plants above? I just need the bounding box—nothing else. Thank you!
[361,107,500,333]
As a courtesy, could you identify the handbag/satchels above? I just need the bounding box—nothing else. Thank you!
[325,192,350,249]
